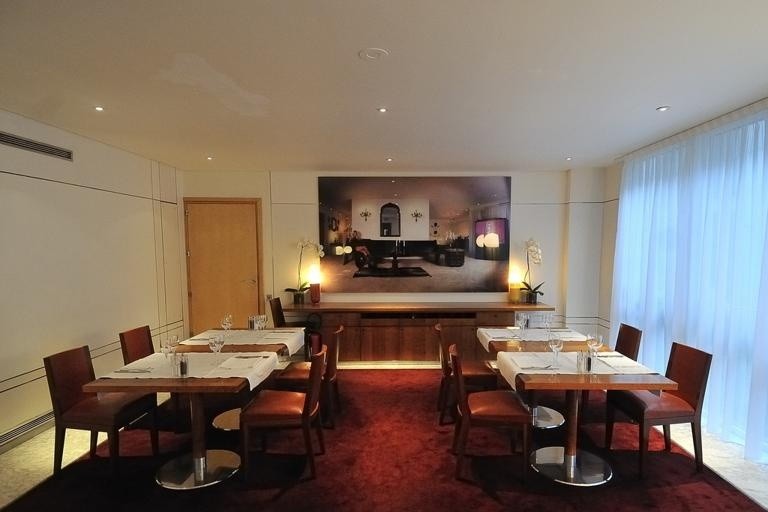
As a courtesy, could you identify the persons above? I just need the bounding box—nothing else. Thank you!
[351,230,372,257]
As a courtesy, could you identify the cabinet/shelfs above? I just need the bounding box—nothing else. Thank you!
[279,297,557,365]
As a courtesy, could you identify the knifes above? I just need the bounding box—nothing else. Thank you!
[235,354,269,359]
[187,337,208,341]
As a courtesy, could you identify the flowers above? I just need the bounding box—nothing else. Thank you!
[282,237,325,294]
[520,235,546,296]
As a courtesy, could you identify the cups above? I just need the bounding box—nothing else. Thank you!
[310,283,321,304]
[510,283,522,305]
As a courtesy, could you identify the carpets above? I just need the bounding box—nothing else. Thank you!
[0,366,767,510]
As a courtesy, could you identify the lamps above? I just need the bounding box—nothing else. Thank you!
[508,281,522,304]
[309,278,322,305]
[359,207,373,222]
[411,210,423,223]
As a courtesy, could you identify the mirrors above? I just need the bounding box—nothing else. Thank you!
[379,202,402,237]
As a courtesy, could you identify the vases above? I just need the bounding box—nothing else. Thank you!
[293,290,306,305]
[525,289,538,305]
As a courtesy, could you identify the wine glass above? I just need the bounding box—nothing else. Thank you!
[516,311,605,374]
[207,333,225,369]
[247,314,269,339]
[218,311,235,338]
[158,334,188,378]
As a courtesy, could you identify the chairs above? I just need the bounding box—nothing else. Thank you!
[40,340,160,485]
[603,339,714,484]
[434,319,502,426]
[117,323,182,434]
[443,342,538,496]
[265,323,349,428]
[266,296,314,363]
[231,342,334,488]
[564,321,647,417]
